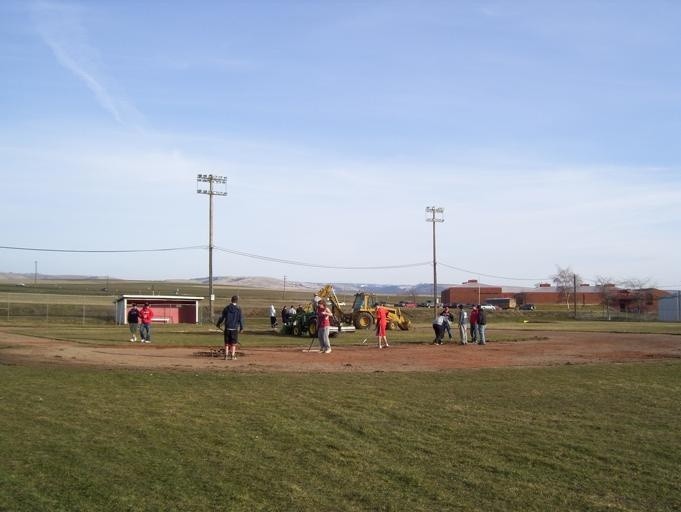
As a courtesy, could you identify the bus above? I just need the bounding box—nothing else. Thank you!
[483,296,516,310]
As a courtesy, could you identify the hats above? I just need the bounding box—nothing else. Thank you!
[131,302,150,309]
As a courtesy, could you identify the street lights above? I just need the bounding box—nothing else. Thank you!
[425,205,446,320]
[196,173,229,323]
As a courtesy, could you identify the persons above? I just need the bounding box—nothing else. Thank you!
[217,295,244,361]
[127,303,140,342]
[316,300,333,354]
[432,304,487,345]
[269,293,321,328]
[373,303,390,349]
[139,302,154,343]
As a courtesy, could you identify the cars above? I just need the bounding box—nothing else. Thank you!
[98,288,108,291]
[14,282,25,286]
[394,301,538,311]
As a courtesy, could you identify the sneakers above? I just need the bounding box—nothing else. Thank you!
[231,354,238,360]
[319,349,331,353]
[458,340,487,345]
[129,334,151,344]
[433,340,443,345]
[378,344,389,349]
[223,355,228,360]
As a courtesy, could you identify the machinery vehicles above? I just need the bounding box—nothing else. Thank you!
[304,284,412,331]
[278,306,341,338]
